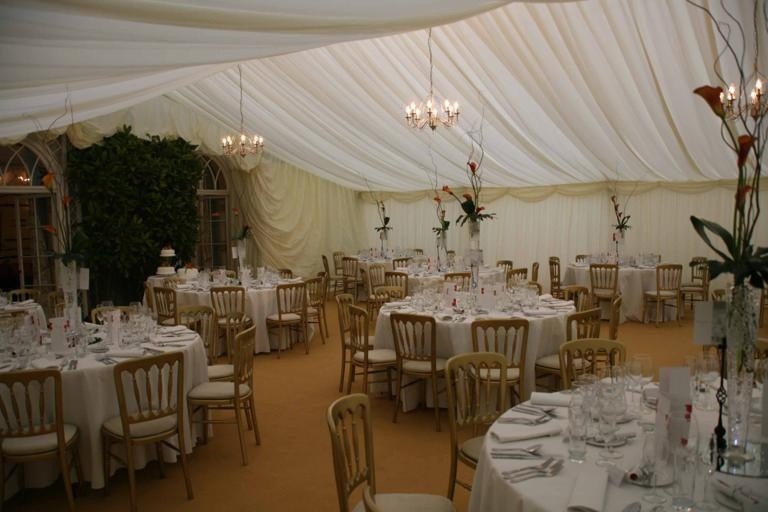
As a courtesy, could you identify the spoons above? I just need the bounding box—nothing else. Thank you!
[493,443,543,452]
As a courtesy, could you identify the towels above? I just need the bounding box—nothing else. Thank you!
[31,358,58,369]
[104,348,144,358]
[488,423,563,442]
[150,334,196,342]
[567,464,611,508]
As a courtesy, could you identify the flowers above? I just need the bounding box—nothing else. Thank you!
[440,108,498,225]
[362,174,394,238]
[598,162,648,236]
[683,0,768,380]
[421,142,450,235]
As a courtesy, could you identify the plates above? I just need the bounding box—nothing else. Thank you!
[632,479,674,486]
[587,439,627,447]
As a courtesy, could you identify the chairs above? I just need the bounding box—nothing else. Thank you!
[443,352,508,500]
[9,288,72,318]
[0,367,77,512]
[326,393,454,512]
[186,325,261,466]
[100,351,194,512]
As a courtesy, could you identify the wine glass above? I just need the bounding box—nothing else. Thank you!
[664,411,699,511]
[624,362,641,403]
[628,354,654,391]
[683,356,697,403]
[600,366,626,398]
[597,409,617,467]
[100,301,115,316]
[96,303,104,330]
[696,352,720,411]
[567,373,599,462]
[641,436,669,503]
[129,302,143,322]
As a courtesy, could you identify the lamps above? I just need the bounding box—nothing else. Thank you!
[406,22,461,129]
[221,63,265,158]
[718,2,768,118]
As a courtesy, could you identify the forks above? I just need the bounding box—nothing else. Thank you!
[522,400,556,412]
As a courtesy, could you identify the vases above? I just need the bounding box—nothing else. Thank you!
[616,231,628,259]
[380,231,389,256]
[723,283,763,458]
[436,232,447,271]
[468,221,481,261]
